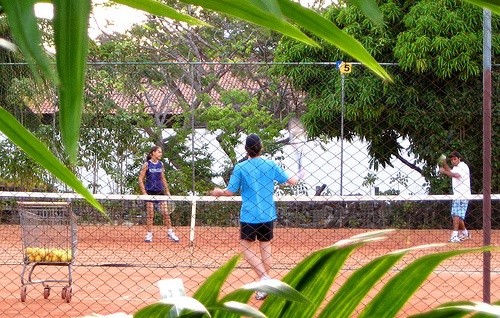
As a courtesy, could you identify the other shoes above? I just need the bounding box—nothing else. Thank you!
[459,230,471,241]
[448,235,460,243]
[255,275,272,300]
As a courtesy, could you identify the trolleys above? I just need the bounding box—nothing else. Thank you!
[15,199,79,304]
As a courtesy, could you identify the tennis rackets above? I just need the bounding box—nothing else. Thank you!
[152,201,176,215]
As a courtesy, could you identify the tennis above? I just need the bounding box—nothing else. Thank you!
[25,247,72,263]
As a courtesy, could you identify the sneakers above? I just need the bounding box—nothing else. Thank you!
[145,234,152,242]
[167,232,178,242]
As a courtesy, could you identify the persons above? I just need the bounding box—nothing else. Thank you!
[212,133,296,299]
[439,151,472,243]
[139,146,180,241]
